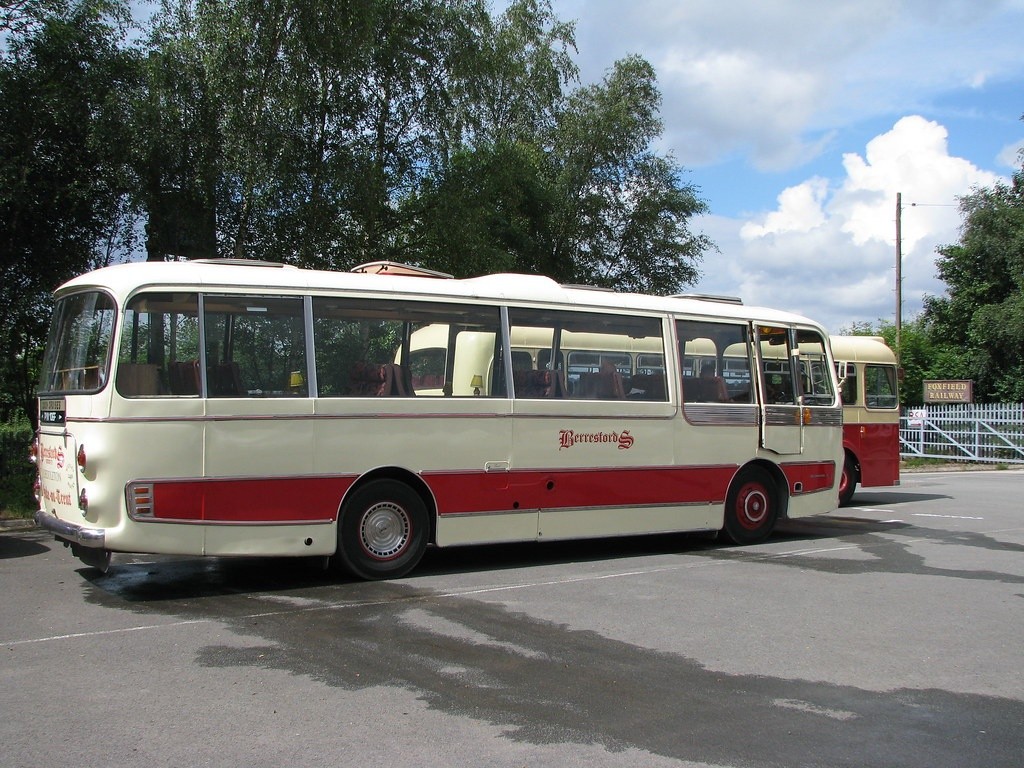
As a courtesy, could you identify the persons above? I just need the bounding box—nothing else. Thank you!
[334,362,376,395]
[699,365,715,378]
[621,378,647,398]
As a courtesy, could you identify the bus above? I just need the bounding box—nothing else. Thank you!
[30,257,845,581]
[384,321,901,510]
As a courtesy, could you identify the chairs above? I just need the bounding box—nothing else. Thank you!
[117,358,805,404]
